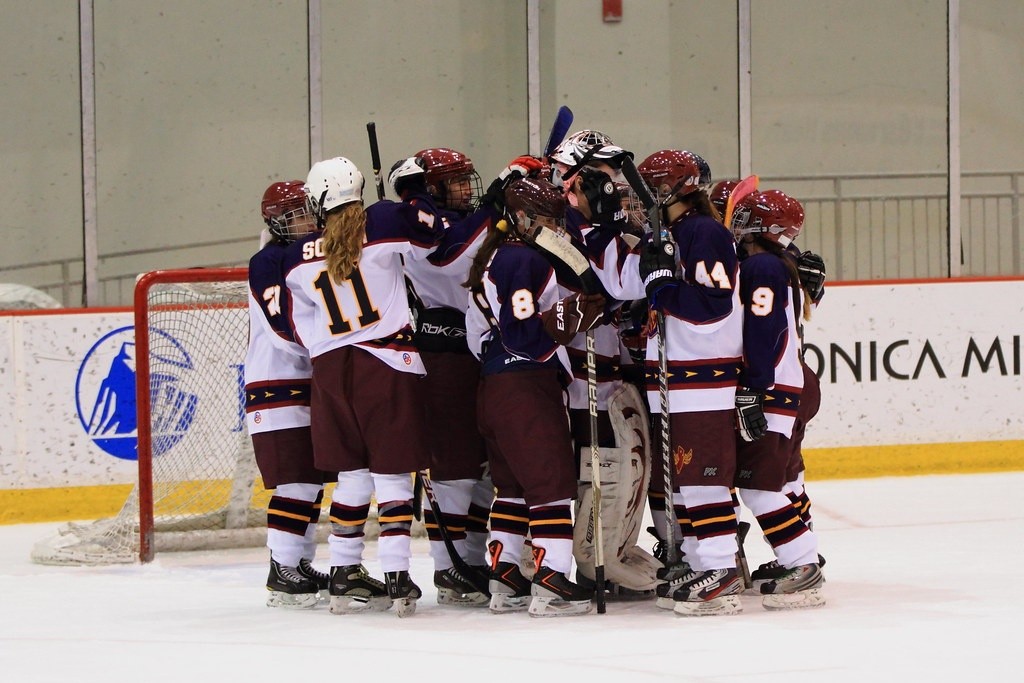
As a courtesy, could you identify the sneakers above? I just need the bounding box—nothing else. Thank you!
[265,526,825,614]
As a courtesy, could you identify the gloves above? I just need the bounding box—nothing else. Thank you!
[620,328,648,368]
[580,170,631,231]
[543,291,607,345]
[389,155,427,198]
[733,385,768,441]
[640,238,677,306]
[486,153,543,200]
[797,250,825,300]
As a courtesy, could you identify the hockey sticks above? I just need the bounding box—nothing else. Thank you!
[619,158,676,565]
[413,458,490,599]
[534,227,608,614]
[364,124,423,333]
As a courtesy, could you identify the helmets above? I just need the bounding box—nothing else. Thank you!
[694,152,711,192]
[710,180,760,222]
[260,180,319,244]
[615,182,649,231]
[729,189,800,252]
[790,197,805,235]
[503,176,567,252]
[303,156,366,231]
[415,149,485,214]
[630,147,701,233]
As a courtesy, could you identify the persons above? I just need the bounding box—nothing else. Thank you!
[248,130,827,617]
